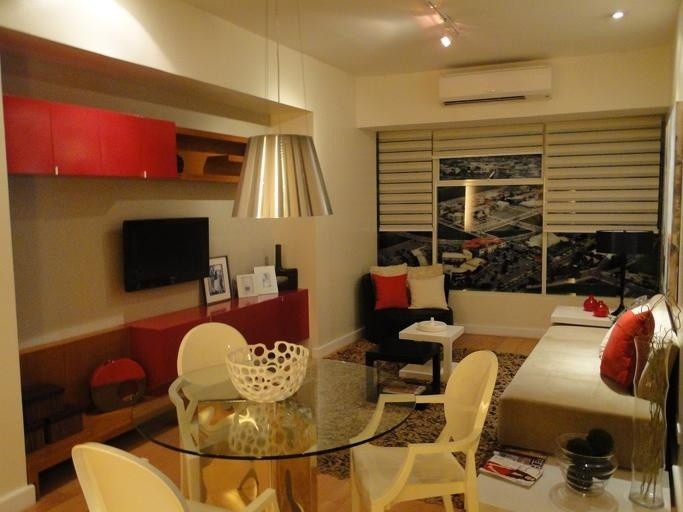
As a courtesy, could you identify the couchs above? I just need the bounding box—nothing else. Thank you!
[498,293,680,473]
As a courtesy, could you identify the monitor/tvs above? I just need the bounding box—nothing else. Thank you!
[122,217,210,293]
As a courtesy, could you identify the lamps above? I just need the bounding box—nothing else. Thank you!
[596,229,654,316]
[232,1,334,220]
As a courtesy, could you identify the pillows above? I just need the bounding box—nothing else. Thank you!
[600,304,648,359]
[372,273,406,310]
[601,309,655,386]
[406,264,443,288]
[371,262,408,285]
[407,275,450,310]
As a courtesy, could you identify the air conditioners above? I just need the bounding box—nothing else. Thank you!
[438,62,552,107]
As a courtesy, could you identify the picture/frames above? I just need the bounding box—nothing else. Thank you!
[236,273,256,299]
[203,255,233,305]
[254,265,278,295]
[658,102,683,302]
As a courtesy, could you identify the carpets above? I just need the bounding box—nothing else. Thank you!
[316,336,526,509]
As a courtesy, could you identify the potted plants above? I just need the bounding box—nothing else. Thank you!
[609,284,681,510]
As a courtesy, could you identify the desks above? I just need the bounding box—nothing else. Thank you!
[130,358,416,511]
[476,455,670,512]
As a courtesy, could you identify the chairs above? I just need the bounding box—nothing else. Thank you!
[360,273,454,344]
[169,321,251,502]
[349,350,498,512]
[71,442,280,512]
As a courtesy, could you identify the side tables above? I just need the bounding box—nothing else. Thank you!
[551,305,617,328]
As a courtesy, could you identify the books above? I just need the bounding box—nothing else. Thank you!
[478,448,547,488]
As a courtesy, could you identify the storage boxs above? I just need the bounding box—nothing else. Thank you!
[3,95,99,178]
[103,109,177,177]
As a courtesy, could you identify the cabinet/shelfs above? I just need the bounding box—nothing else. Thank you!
[20,288,309,499]
[178,124,250,182]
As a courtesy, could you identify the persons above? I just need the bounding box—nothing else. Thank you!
[484,461,536,482]
